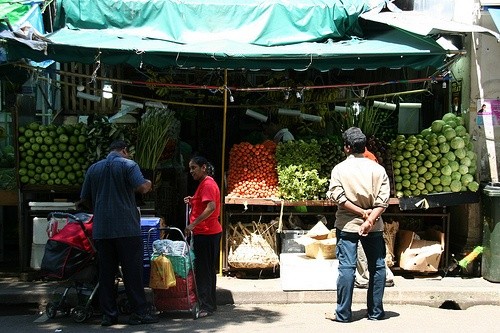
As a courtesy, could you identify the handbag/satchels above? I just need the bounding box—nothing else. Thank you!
[150,255,177,291]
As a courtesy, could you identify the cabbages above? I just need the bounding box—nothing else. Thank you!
[431,113,479,192]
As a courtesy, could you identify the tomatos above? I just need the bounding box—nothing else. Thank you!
[227,140,278,198]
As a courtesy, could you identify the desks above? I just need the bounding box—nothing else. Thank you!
[224,196,450,275]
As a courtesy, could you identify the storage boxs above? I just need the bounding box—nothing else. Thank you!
[33,217,67,245]
[141,218,166,271]
[295,220,338,258]
[32,246,46,269]
[281,253,341,289]
[397,228,445,272]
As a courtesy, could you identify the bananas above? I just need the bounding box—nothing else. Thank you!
[145,69,175,97]
[265,78,343,133]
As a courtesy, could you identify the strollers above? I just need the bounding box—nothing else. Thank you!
[41,212,133,322]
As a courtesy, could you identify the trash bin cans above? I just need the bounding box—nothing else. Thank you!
[480,179,500,284]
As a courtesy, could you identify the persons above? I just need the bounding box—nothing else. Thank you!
[79,139,160,326]
[184,153,223,316]
[354,146,395,287]
[478,104,486,112]
[264,117,295,142]
[167,139,192,228]
[325,127,390,323]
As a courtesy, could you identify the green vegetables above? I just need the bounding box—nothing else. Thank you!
[276,139,327,201]
[134,106,177,168]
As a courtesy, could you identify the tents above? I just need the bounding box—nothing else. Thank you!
[0,0,466,277]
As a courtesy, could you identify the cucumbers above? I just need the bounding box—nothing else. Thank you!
[318,140,346,177]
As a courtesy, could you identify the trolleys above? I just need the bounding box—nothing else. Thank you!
[147,227,202,320]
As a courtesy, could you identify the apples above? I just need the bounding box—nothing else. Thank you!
[17,121,87,184]
[376,112,394,140]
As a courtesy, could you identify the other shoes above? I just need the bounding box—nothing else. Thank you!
[325,312,337,321]
[128,314,158,324]
[199,309,211,318]
[100,317,118,325]
[353,283,369,288]
[385,280,394,287]
